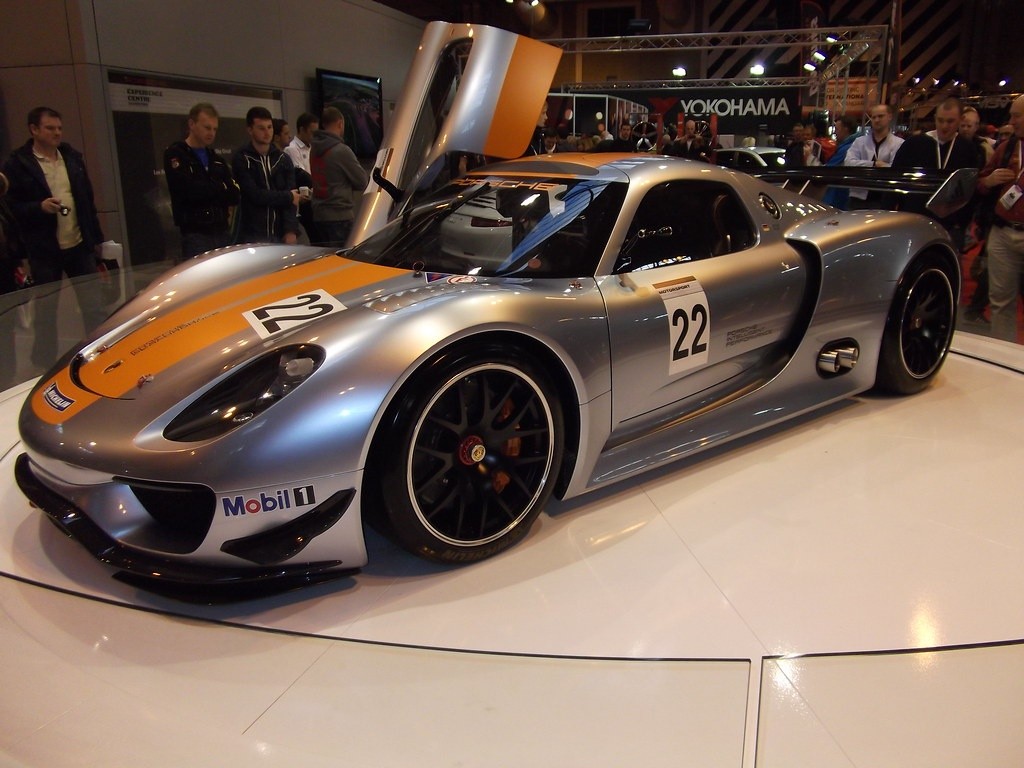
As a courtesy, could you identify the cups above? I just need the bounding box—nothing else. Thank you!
[56,193,73,216]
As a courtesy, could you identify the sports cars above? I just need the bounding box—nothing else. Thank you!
[14,20,994,605]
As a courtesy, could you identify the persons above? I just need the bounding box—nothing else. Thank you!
[731,137,756,170]
[0,106,108,394]
[309,106,370,249]
[283,111,320,175]
[539,120,713,164]
[227,102,310,247]
[272,119,291,151]
[271,118,311,246]
[162,102,242,257]
[450,151,481,181]
[785,94,1024,345]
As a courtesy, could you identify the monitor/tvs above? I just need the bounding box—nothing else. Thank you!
[316,68,383,158]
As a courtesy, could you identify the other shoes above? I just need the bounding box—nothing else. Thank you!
[34,358,55,371]
[960,312,992,328]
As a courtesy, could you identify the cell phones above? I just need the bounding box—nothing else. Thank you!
[689,135,696,140]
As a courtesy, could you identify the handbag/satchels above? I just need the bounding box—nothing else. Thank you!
[96,258,120,307]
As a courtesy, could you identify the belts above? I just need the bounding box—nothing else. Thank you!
[997,214,1024,231]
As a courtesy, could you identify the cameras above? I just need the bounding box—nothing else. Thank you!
[58,202,72,216]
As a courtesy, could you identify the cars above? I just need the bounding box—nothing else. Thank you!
[714,146,790,173]
